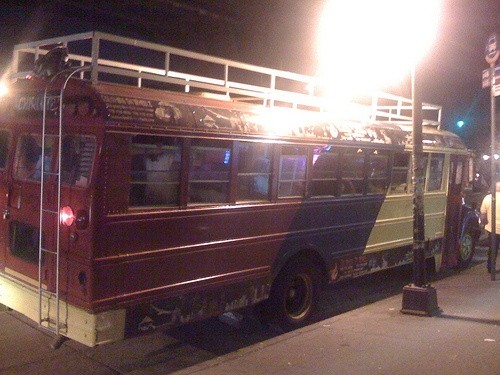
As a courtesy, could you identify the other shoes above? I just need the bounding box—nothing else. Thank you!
[486,264,496,273]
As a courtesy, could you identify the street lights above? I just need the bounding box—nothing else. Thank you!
[395,0,446,318]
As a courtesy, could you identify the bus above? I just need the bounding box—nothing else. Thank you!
[0,29,483,352]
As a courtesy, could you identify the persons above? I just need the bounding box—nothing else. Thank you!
[480,181,500,273]
